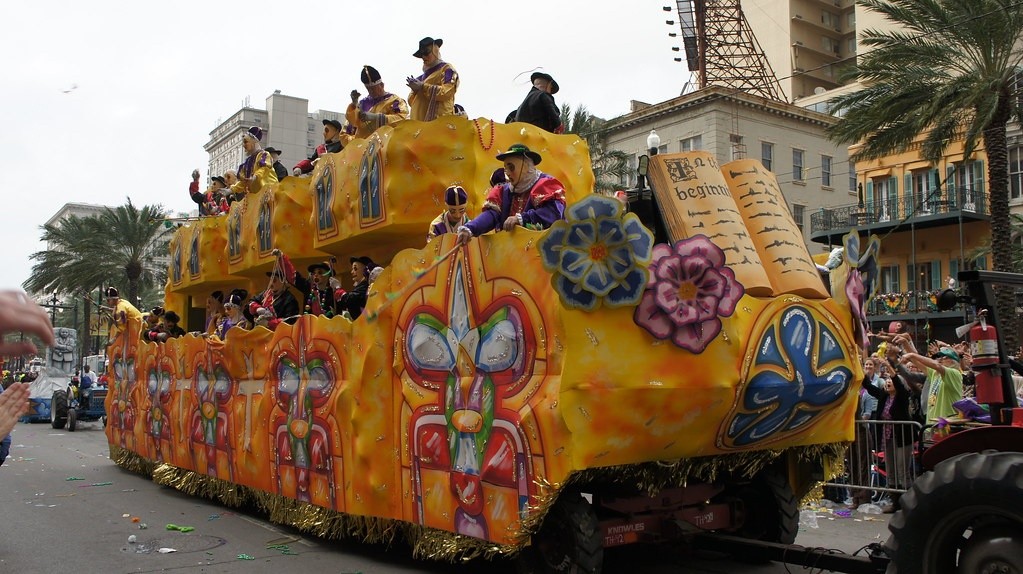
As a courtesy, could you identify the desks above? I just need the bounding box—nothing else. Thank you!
[930,200,955,214]
[850,212,874,225]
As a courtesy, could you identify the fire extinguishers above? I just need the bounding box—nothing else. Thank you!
[969,309,1003,403]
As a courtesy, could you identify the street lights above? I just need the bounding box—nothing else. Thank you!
[45,293,64,327]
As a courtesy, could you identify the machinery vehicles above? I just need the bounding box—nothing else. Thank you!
[50,366,107,432]
[879,269,1023,574]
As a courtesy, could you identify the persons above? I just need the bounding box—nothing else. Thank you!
[99,287,142,330]
[329,255,377,321]
[405,37,461,122]
[426,184,472,244]
[945,275,956,290]
[345,64,410,140]
[0,286,56,467]
[189,126,288,215]
[52,328,75,374]
[455,143,567,247]
[242,270,300,330]
[271,249,346,320]
[822,320,1023,514]
[205,289,249,339]
[77,365,97,407]
[515,71,564,136]
[291,118,342,176]
[338,118,357,146]
[140,305,187,343]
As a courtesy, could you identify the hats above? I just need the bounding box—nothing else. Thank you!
[489,168,510,188]
[531,72,559,94]
[211,176,227,187]
[361,65,381,87]
[413,37,444,58]
[266,270,284,278]
[307,261,336,278]
[322,119,342,133]
[938,349,961,362]
[264,147,282,156]
[211,291,224,303]
[150,307,165,317]
[162,311,180,323]
[223,289,248,309]
[444,185,467,206]
[246,126,263,141]
[495,144,542,165]
[350,256,372,265]
[105,287,120,299]
